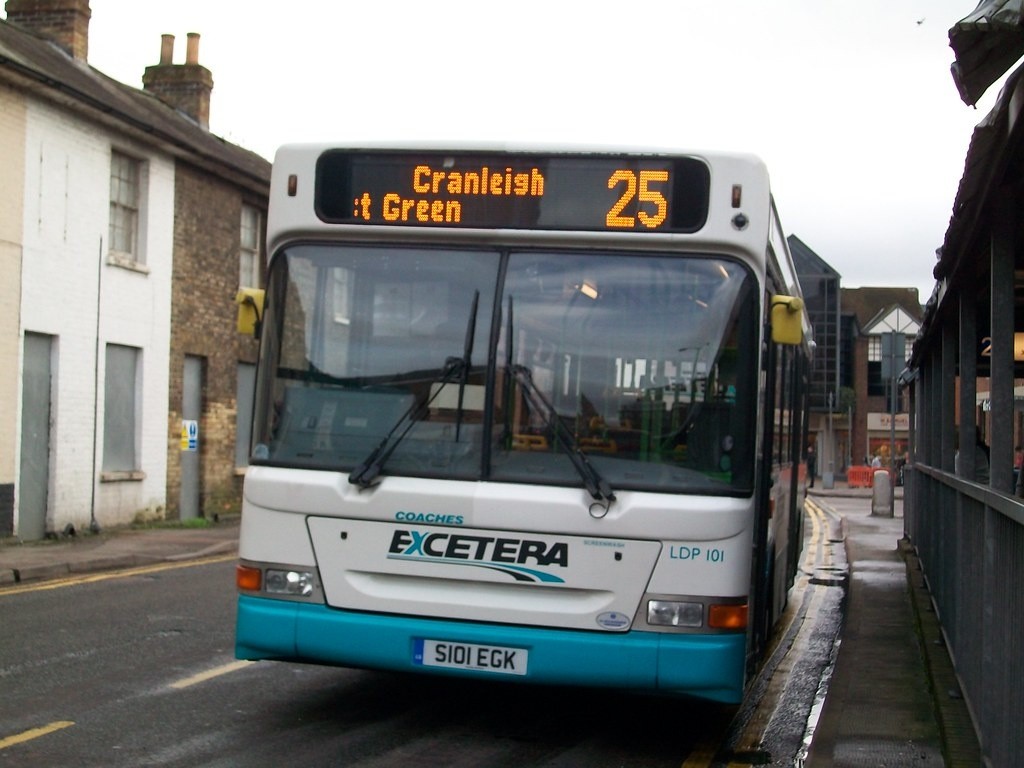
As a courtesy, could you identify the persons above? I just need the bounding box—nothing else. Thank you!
[807,446,816,488]
[899,452,909,486]
[953,423,990,487]
[408,323,486,411]
[1014,446,1023,469]
[871,447,882,468]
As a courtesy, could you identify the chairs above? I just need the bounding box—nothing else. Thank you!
[528,386,691,454]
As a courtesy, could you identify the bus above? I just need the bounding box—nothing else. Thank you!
[235,137,817,706]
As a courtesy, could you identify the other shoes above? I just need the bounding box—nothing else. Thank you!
[809,485,813,488]
